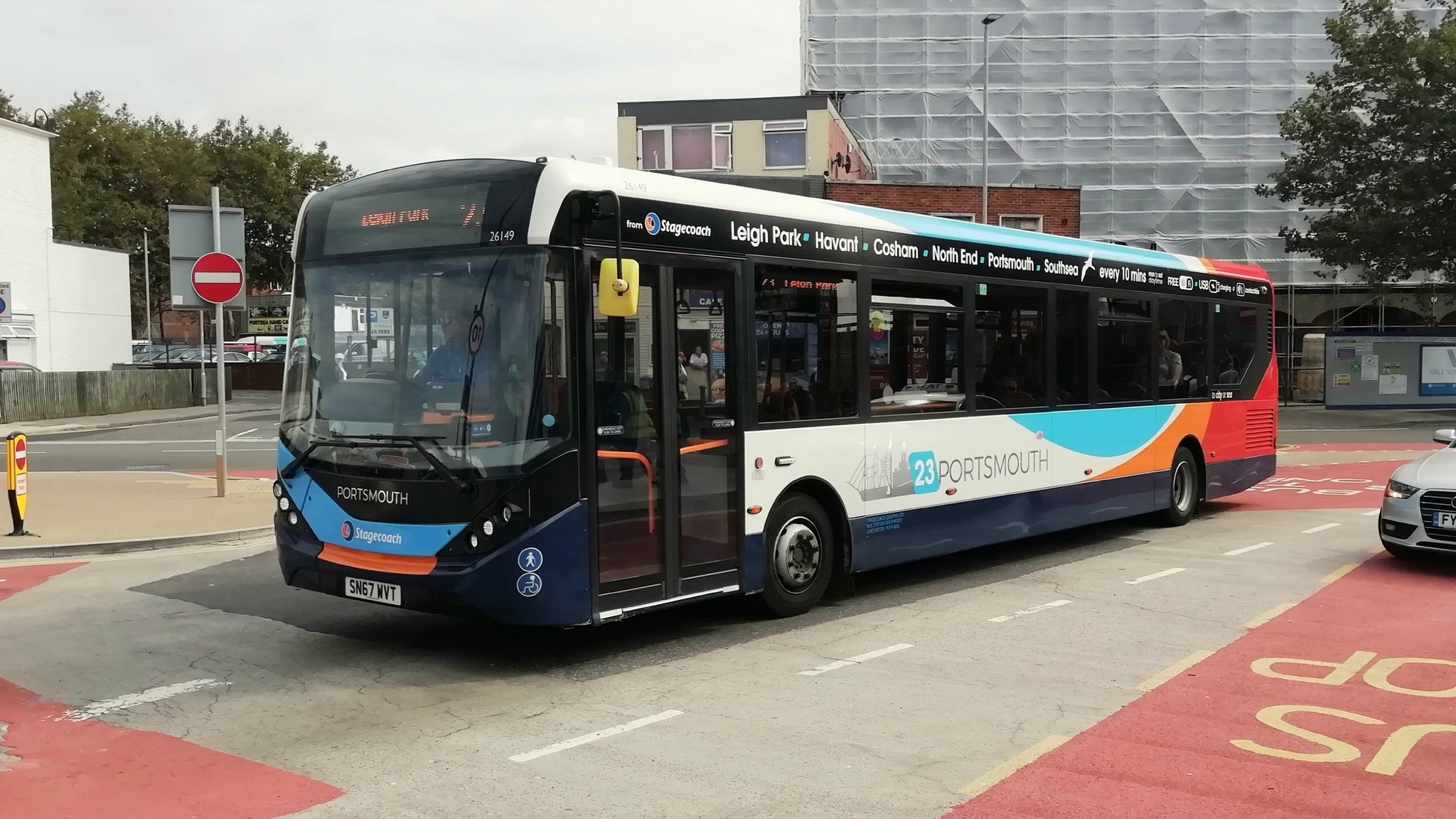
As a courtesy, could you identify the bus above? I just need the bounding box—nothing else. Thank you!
[268,151,1281,634]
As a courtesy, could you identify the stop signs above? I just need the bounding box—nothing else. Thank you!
[15,440,26,469]
[190,251,245,304]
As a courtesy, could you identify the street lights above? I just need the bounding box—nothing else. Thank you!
[979,10,1008,226]
[143,227,155,365]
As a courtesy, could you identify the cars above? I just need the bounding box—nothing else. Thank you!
[1378,429,1456,567]
[0,360,43,373]
[132,333,401,365]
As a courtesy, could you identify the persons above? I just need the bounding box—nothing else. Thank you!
[413,302,501,398]
[1159,327,1182,386]
[679,347,708,400]
[608,369,658,488]
[999,377,1018,397]
